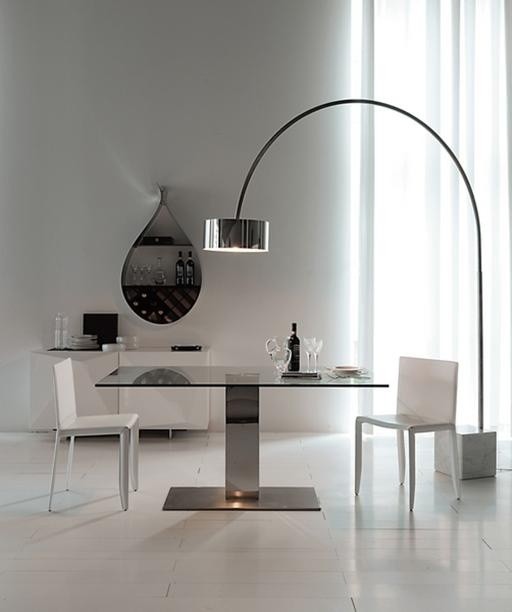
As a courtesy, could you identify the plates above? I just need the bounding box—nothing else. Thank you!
[334,366,360,372]
[67,345,100,350]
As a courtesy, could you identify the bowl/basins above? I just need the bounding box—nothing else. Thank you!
[102,344,122,352]
[69,335,98,346]
[116,336,136,348]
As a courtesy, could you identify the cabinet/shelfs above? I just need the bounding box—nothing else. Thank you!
[120,185,203,325]
[28,347,212,440]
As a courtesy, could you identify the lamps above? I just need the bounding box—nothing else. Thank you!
[204,98,496,481]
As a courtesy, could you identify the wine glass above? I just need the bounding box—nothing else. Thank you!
[301,336,316,373]
[131,264,152,285]
[311,337,323,373]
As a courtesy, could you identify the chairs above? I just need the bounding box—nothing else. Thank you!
[48,358,139,511]
[355,357,460,511]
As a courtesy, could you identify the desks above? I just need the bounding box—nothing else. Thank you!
[94,366,389,511]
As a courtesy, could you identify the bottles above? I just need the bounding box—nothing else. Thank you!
[186,251,195,285]
[154,256,168,285]
[54,311,68,350]
[175,251,186,286]
[287,323,300,371]
[133,293,164,315]
